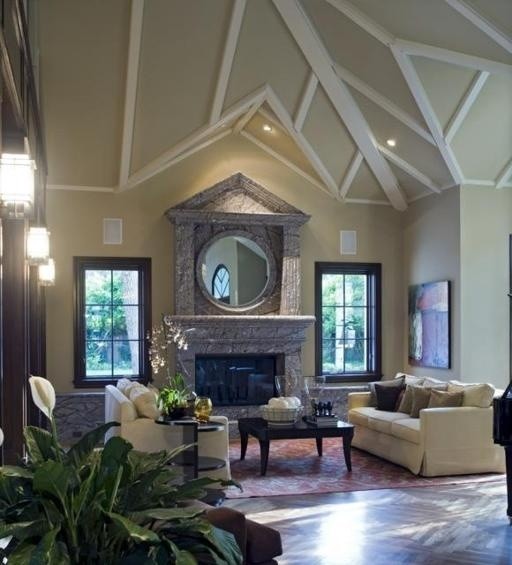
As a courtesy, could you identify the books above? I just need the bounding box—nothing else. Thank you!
[305,413,340,427]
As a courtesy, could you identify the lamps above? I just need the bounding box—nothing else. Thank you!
[0,153,59,289]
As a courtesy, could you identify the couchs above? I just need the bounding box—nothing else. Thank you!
[348,369,510,474]
[103,378,281,565]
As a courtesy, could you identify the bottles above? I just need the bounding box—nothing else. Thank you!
[195,396,213,421]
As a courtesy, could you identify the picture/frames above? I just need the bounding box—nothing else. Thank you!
[407,278,451,371]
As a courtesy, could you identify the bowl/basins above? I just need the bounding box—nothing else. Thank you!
[260,404,304,426]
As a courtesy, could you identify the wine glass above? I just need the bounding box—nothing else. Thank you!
[274,374,297,397]
[303,376,325,401]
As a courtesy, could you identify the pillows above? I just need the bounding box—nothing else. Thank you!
[368,375,495,418]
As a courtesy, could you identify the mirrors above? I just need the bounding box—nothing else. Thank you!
[195,228,278,312]
